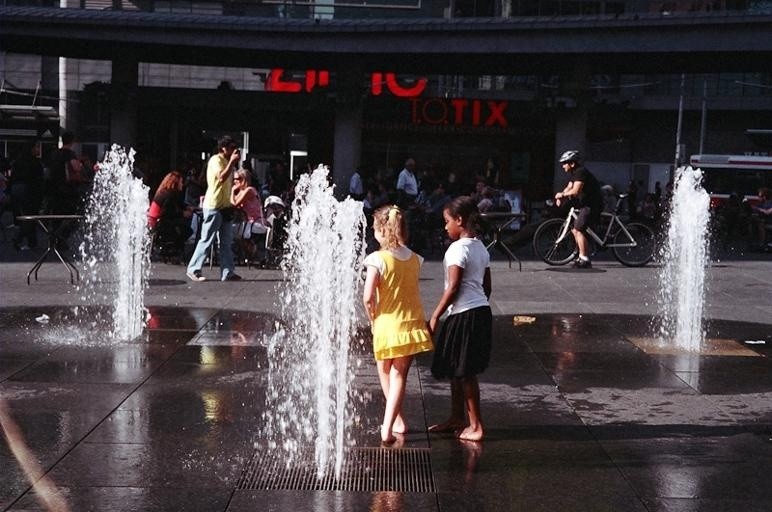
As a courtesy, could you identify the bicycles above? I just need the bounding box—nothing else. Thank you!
[534,191,658,267]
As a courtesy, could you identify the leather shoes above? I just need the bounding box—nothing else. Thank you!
[569,257,580,267]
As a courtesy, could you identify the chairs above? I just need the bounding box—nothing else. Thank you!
[150,203,288,267]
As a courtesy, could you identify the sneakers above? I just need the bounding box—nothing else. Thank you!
[575,259,592,269]
[221,274,242,282]
[186,272,207,282]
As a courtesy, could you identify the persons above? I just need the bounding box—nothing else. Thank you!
[346,146,509,253]
[553,148,601,269]
[363,203,434,443]
[149,167,272,263]
[423,196,492,443]
[186,134,242,282]
[623,178,771,252]
[8,132,99,248]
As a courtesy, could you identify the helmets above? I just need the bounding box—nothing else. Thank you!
[559,150,579,164]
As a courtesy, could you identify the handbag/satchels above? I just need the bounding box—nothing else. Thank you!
[266,212,292,251]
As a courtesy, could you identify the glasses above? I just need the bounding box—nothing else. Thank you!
[232,177,241,181]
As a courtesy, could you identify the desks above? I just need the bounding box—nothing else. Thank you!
[13,212,90,287]
[473,212,531,271]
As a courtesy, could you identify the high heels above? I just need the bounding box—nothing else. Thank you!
[171,255,181,264]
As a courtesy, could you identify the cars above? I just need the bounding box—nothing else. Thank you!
[0,174,7,205]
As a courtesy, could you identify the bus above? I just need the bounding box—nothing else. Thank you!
[688,154,772,235]
